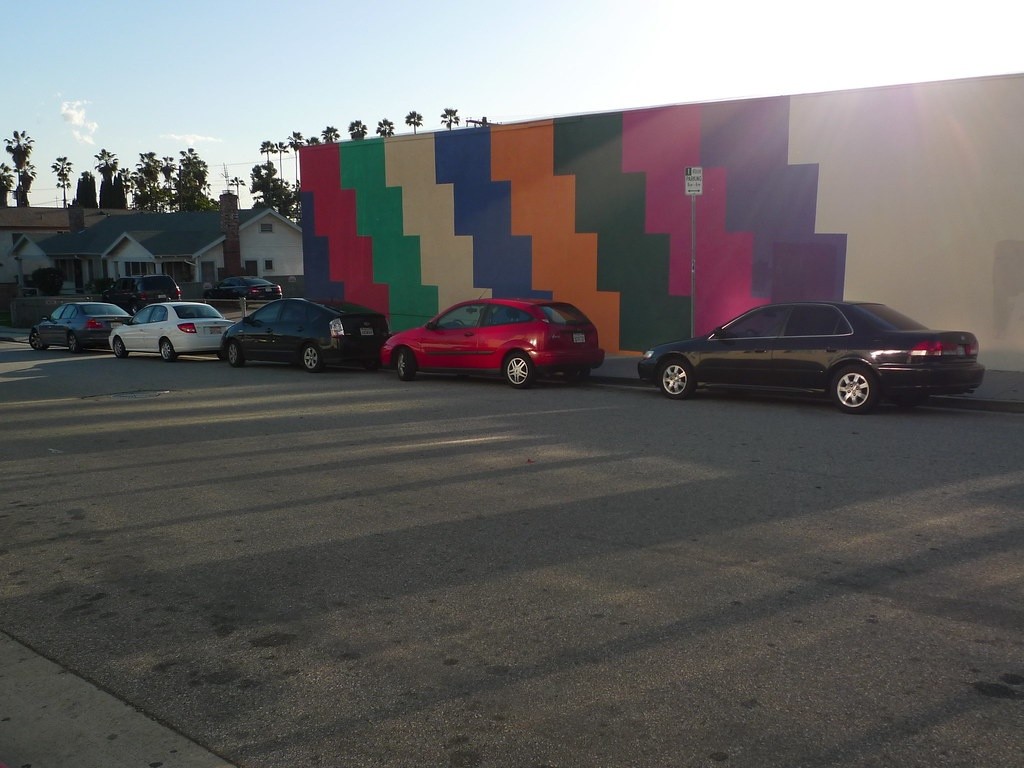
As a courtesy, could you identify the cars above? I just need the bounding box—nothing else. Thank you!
[27,304,133,355]
[200,275,282,310]
[220,298,389,376]
[106,301,234,362]
[636,297,986,412]
[380,294,605,388]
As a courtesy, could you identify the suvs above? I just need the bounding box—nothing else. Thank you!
[103,274,181,316]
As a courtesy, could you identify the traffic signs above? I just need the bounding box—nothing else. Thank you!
[683,162,705,199]
